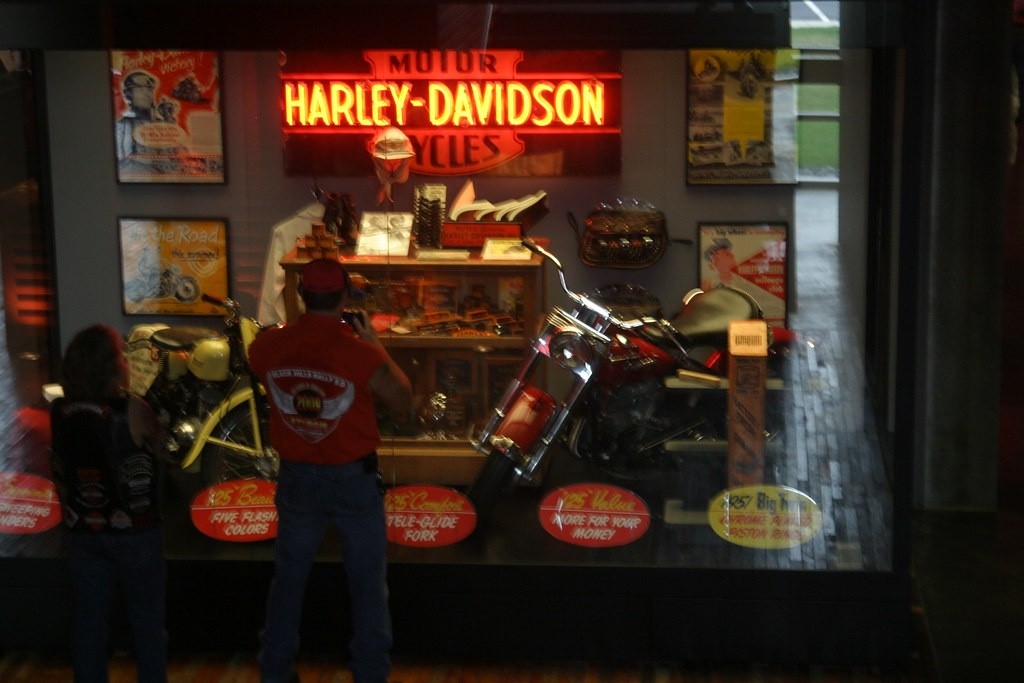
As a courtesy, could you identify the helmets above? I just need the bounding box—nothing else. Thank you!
[187,338,233,381]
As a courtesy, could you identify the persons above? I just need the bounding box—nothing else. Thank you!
[48,321,175,683]
[248,259,416,682]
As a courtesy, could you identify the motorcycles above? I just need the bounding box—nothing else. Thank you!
[43,223,786,507]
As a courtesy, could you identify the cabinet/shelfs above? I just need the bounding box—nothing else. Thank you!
[278,238,547,488]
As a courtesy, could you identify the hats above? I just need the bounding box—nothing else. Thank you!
[366,126,416,160]
[299,257,349,293]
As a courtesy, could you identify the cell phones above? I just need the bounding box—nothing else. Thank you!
[341,312,363,330]
